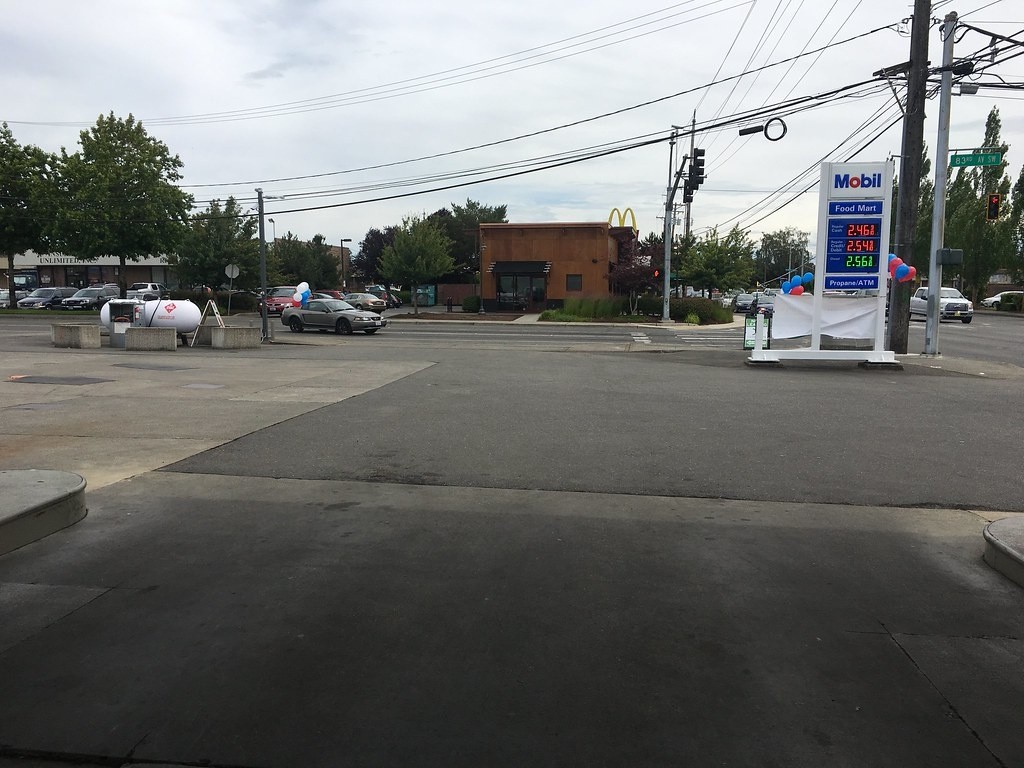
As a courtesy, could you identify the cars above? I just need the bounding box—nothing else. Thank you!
[371,291,403,308]
[731,294,755,313]
[233,291,263,304]
[749,297,774,318]
[315,290,346,300]
[751,289,813,304]
[0,291,28,310]
[281,299,388,336]
[670,285,746,309]
[342,293,386,315]
[311,293,335,299]
[258,286,313,317]
[980,291,1024,310]
[257,288,273,296]
[126,282,172,302]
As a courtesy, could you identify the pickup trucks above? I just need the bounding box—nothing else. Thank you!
[907,287,974,324]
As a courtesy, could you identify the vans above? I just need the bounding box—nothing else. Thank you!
[17,287,79,311]
[93,282,121,299]
[4,273,38,292]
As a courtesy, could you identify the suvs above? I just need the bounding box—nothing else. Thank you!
[61,287,118,312]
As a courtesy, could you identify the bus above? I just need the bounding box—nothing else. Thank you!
[193,286,244,297]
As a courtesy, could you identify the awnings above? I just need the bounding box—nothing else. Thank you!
[486,260,553,273]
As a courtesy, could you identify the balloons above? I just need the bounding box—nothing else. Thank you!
[899,266,917,282]
[791,275,802,288]
[789,286,804,295]
[802,272,814,284]
[292,281,311,307]
[895,262,910,280]
[890,257,904,278]
[888,272,893,279]
[782,281,792,294]
[888,253,897,272]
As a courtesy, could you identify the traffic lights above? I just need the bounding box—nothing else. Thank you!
[986,194,1001,220]
[654,269,662,278]
[683,179,693,203]
[689,148,705,190]
